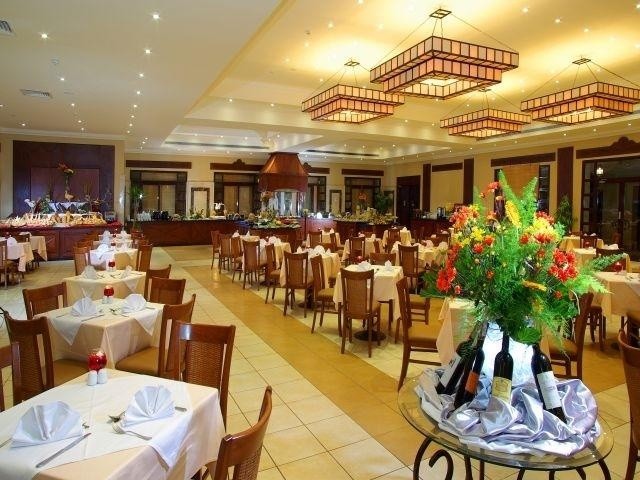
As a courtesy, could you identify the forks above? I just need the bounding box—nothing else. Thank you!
[112,423,153,440]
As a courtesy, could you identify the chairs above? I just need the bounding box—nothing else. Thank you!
[214,385,272,480]
[617,329,640,480]
[547,290,594,381]
[396,276,444,391]
[174,319,236,480]
[0,228,196,412]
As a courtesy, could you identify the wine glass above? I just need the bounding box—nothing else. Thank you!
[357,255,363,264]
[614,261,622,275]
[585,241,590,250]
[88,229,131,374]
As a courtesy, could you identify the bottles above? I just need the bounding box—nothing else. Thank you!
[366,206,371,220]
[436,318,567,425]
[356,205,360,219]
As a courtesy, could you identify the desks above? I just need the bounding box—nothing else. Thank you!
[397,370,614,480]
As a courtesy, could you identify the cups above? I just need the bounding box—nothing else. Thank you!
[385,262,392,270]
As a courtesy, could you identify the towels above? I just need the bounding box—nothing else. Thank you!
[123,383,176,427]
[9,401,82,448]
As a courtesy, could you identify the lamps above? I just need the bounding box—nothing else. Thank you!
[520,56,640,126]
[370,8,520,100]
[440,87,532,139]
[302,57,405,126]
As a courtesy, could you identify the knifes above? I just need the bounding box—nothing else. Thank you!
[36,433,98,468]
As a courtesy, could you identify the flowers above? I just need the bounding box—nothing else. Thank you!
[420,170,624,364]
